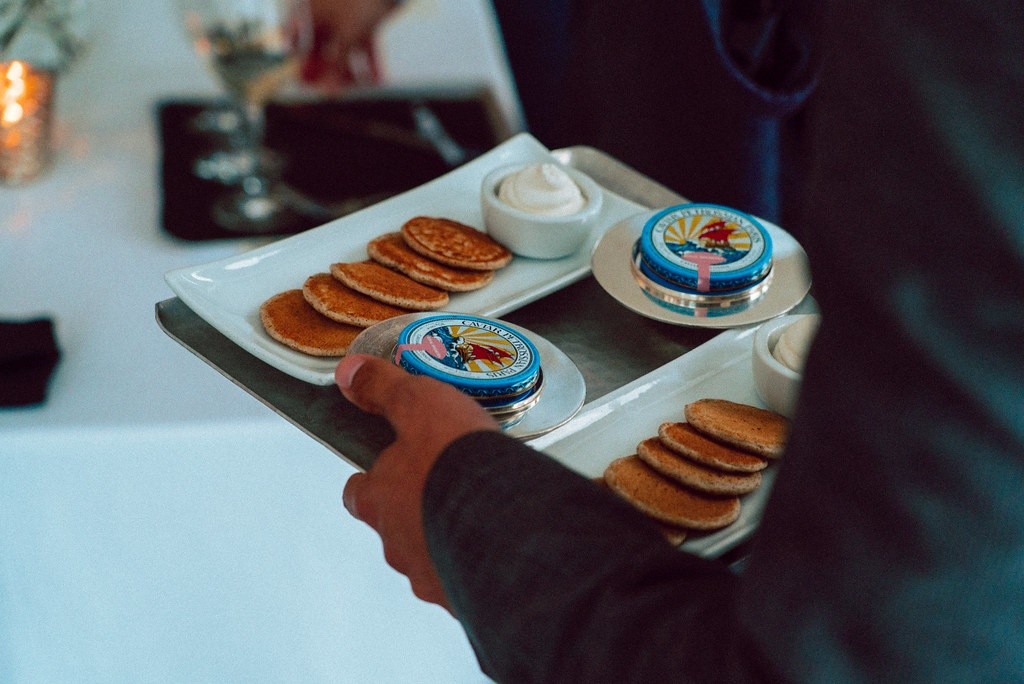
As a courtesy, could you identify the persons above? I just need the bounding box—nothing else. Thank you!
[344,1,1024,681]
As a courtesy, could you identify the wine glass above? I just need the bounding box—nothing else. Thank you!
[182,0,282,179]
[202,0,310,229]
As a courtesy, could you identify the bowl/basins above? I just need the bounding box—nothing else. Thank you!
[752,314,822,419]
[481,161,603,260]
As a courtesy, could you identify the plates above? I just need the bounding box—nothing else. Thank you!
[350,309,587,443]
[524,294,824,559]
[593,208,812,328]
[162,130,649,386]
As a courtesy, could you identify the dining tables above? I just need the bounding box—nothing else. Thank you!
[0,0,605,684]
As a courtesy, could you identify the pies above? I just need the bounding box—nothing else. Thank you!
[259,217,513,357]
[591,398,793,549]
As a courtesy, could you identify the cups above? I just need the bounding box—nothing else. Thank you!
[3,55,58,184]
